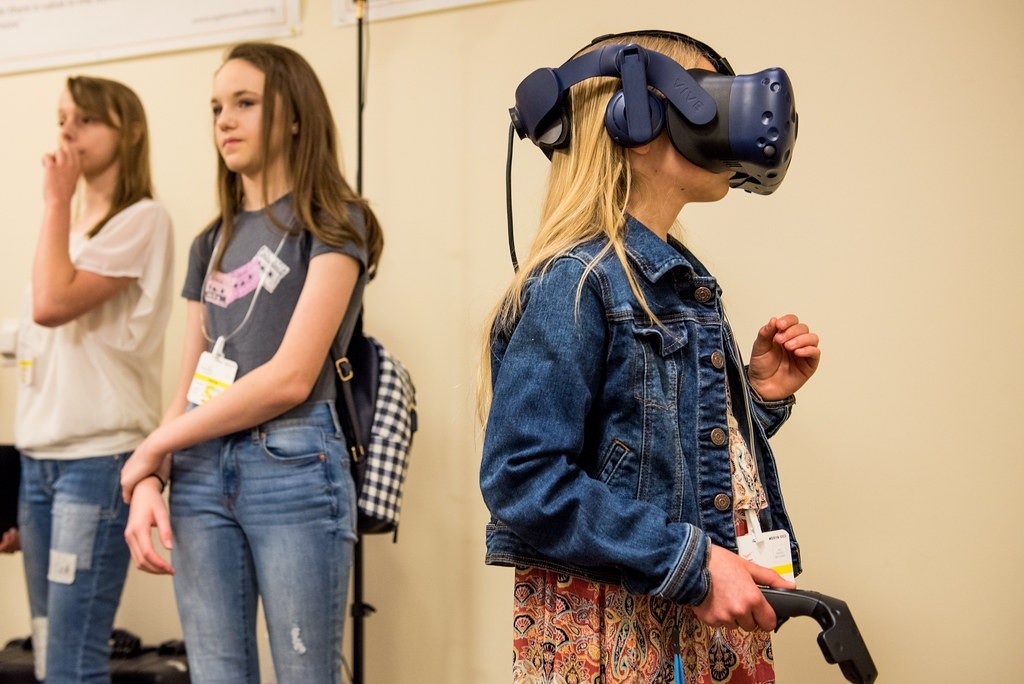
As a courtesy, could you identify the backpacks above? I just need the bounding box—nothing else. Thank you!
[204,205,418,545]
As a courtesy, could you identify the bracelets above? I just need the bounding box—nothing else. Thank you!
[150,473,164,493]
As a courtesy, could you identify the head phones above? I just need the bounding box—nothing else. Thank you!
[605,44,666,147]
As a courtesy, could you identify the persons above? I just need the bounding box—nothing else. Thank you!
[0,76,173,684]
[478,31,820,684]
[121,44,381,684]
